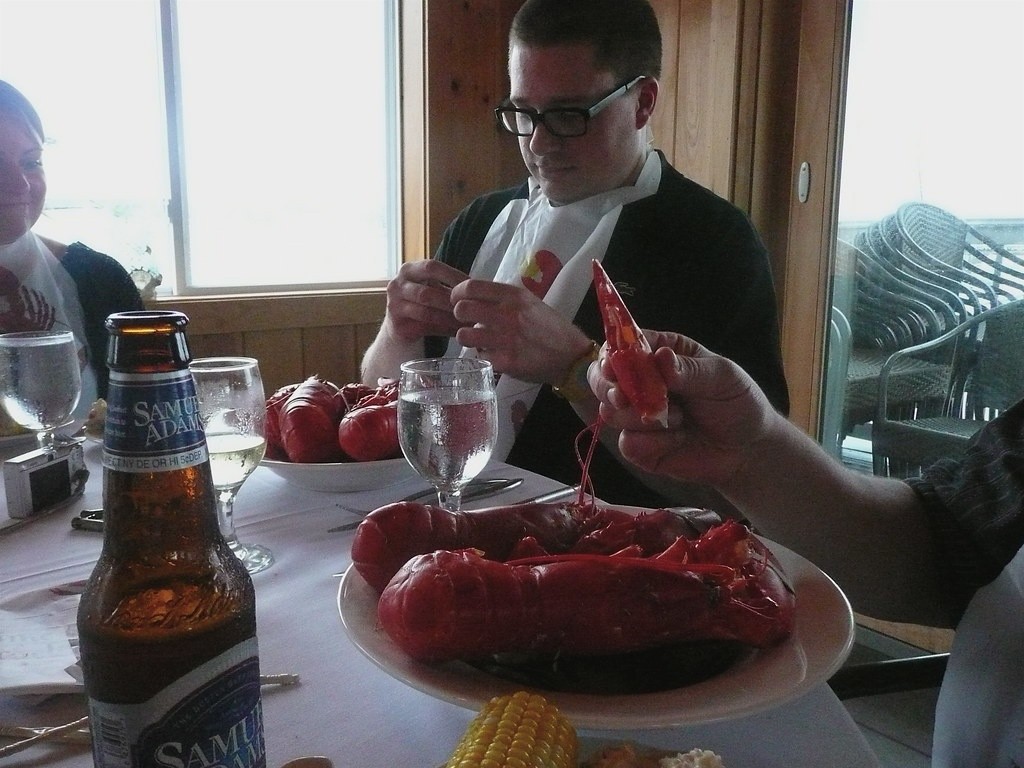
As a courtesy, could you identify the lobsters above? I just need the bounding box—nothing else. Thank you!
[338,376,487,462]
[350,259,724,592]
[224,388,279,457]
[279,374,338,463]
[378,519,793,669]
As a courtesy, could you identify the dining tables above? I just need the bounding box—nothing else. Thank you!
[0,433,880,768]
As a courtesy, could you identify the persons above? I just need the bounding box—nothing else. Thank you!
[0,80,149,427]
[358,0,790,530]
[585,327,1024,630]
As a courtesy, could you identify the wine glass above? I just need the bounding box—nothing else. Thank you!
[0,330,82,449]
[397,357,498,512]
[188,356,273,575]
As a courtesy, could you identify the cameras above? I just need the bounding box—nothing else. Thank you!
[4,442,88,519]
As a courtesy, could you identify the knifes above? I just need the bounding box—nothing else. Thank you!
[326,477,525,534]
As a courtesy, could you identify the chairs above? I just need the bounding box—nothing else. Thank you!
[818,201,1023,480]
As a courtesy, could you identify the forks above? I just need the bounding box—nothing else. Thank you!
[336,478,510,516]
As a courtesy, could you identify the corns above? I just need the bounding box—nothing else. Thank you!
[444,691,577,768]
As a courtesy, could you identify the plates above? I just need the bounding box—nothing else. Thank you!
[259,458,418,492]
[337,531,855,730]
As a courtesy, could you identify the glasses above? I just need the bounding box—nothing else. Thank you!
[494,75,648,137]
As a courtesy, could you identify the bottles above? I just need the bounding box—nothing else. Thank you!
[76,308,267,768]
